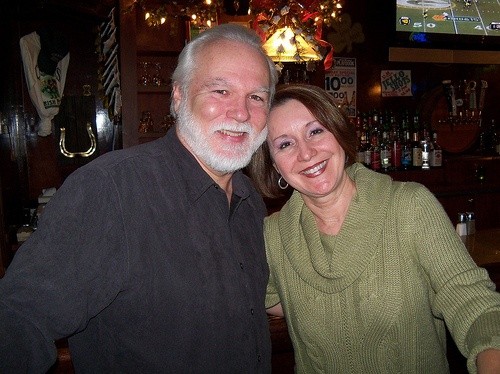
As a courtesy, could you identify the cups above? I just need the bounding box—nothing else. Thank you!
[0,112,37,138]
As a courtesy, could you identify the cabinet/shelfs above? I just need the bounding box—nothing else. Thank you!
[7,20,99,199]
[120,0,325,149]
[347,47,500,229]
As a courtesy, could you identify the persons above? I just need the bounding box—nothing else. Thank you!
[262,83,500,374]
[0,24,278,374]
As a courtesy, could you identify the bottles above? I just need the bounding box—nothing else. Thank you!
[456,213,467,236]
[466,212,475,235]
[357,112,442,172]
[477,119,499,156]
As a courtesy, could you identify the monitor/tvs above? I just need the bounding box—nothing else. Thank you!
[395,0,500,51]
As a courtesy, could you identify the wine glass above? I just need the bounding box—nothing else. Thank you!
[140,112,173,134]
[137,61,177,89]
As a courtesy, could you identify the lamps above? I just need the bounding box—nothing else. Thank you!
[262,0,325,61]
